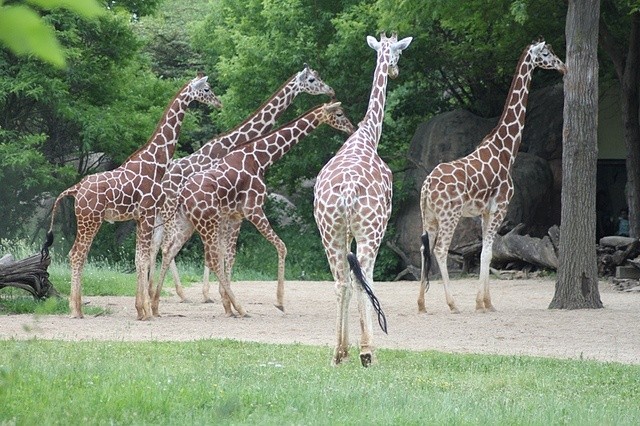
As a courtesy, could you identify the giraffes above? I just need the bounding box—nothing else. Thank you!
[313,31,413,368]
[41,69,223,321]
[149,63,334,304]
[417,34,568,315]
[154,95,359,318]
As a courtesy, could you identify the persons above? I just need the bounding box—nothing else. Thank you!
[616,208,629,238]
